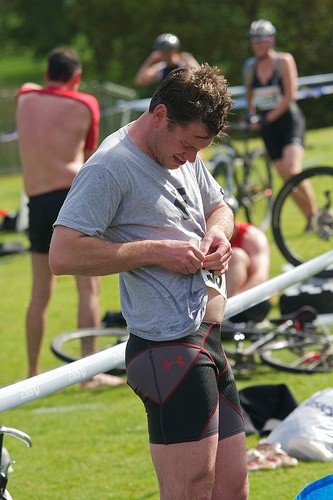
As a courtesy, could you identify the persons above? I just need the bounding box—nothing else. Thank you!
[133,31,202,87]
[241,18,319,234]
[13,45,129,392]
[223,197,270,323]
[49,61,252,499]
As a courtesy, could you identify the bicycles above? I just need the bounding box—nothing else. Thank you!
[197,117,276,230]
[268,165,333,267]
[53,307,333,375]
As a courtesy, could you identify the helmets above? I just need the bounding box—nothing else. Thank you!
[250,18,278,36]
[156,33,178,50]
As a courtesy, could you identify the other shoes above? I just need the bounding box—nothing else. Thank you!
[254,442,298,466]
[246,448,275,472]
